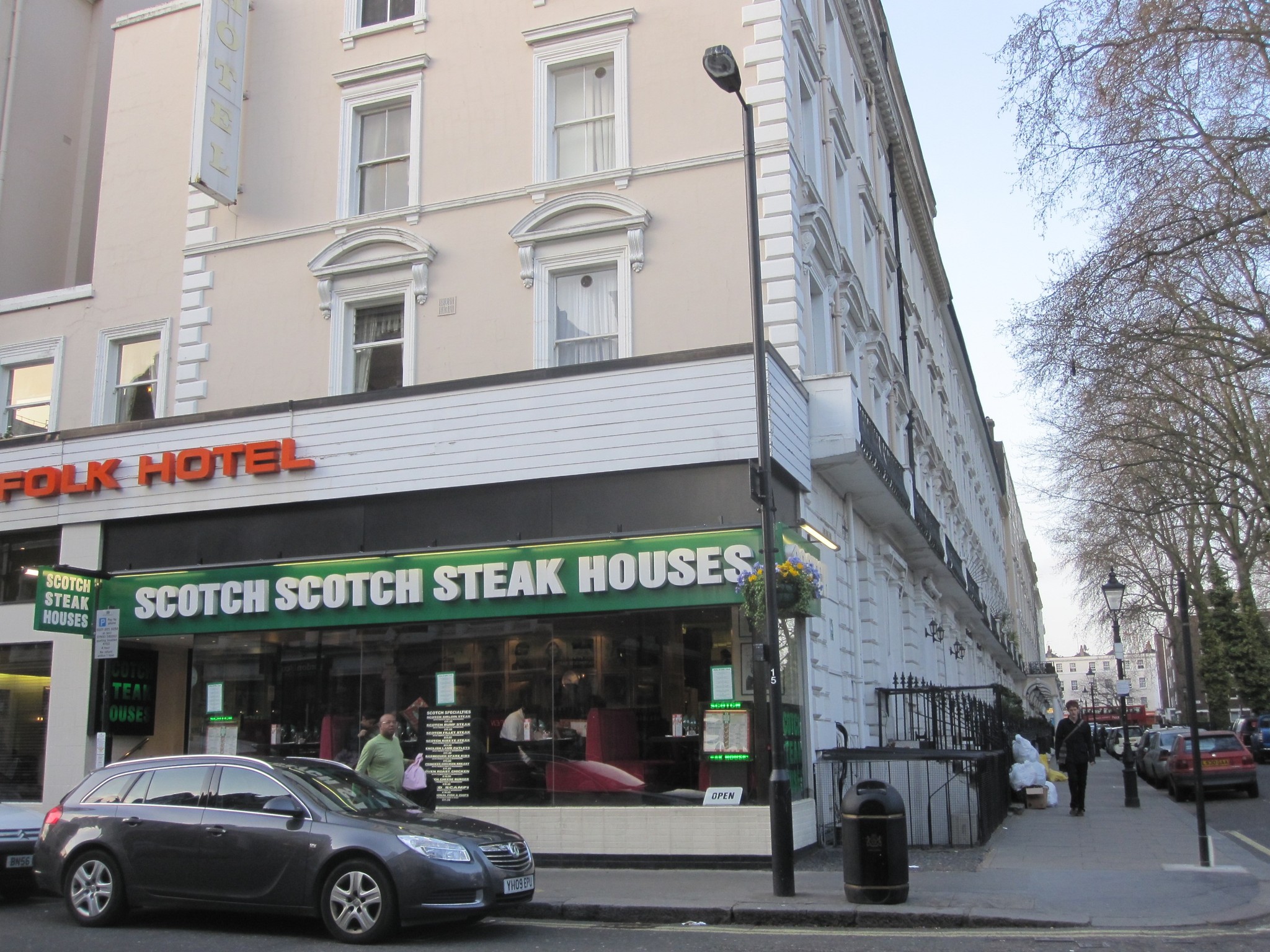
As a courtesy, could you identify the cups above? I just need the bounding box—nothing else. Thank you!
[544,730,550,738]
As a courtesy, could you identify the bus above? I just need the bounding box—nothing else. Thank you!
[1081,704,1147,731]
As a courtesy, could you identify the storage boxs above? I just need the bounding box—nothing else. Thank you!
[1024,785,1049,809]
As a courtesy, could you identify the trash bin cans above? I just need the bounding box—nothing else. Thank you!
[840,780,910,904]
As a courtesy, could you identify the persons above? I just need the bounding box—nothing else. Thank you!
[1166,719,1173,728]
[1055,700,1095,816]
[348,711,416,794]
[1098,724,1107,749]
[499,703,547,788]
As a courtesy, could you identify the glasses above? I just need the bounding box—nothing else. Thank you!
[383,721,397,724]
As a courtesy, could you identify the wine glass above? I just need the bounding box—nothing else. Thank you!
[406,725,413,741]
[397,724,405,741]
[532,723,545,740]
[682,715,697,736]
[280,728,319,743]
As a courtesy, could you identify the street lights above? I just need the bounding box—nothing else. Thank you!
[1082,686,1089,723]
[703,42,796,896]
[1086,666,1101,757]
[1078,698,1084,720]
[1100,566,1140,807]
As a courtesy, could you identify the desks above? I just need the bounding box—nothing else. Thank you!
[251,741,320,758]
[489,736,575,757]
[641,735,701,747]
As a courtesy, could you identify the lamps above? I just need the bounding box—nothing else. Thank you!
[21,566,38,577]
[956,644,966,660]
[933,624,945,644]
[949,639,961,658]
[797,517,841,553]
[1032,686,1050,710]
[924,617,938,636]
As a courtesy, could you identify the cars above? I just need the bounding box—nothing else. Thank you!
[1086,713,1218,791]
[465,750,707,803]
[1159,730,1259,804]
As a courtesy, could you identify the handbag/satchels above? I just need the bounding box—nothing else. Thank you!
[401,752,428,791]
[1056,742,1067,764]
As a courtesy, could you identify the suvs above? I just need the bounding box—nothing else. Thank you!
[30,750,539,946]
[1249,714,1270,764]
[1228,715,1259,749]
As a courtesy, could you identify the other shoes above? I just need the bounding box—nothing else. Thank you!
[1070,807,1078,815]
[1078,808,1084,816]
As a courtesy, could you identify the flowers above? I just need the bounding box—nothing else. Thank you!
[733,554,824,636]
